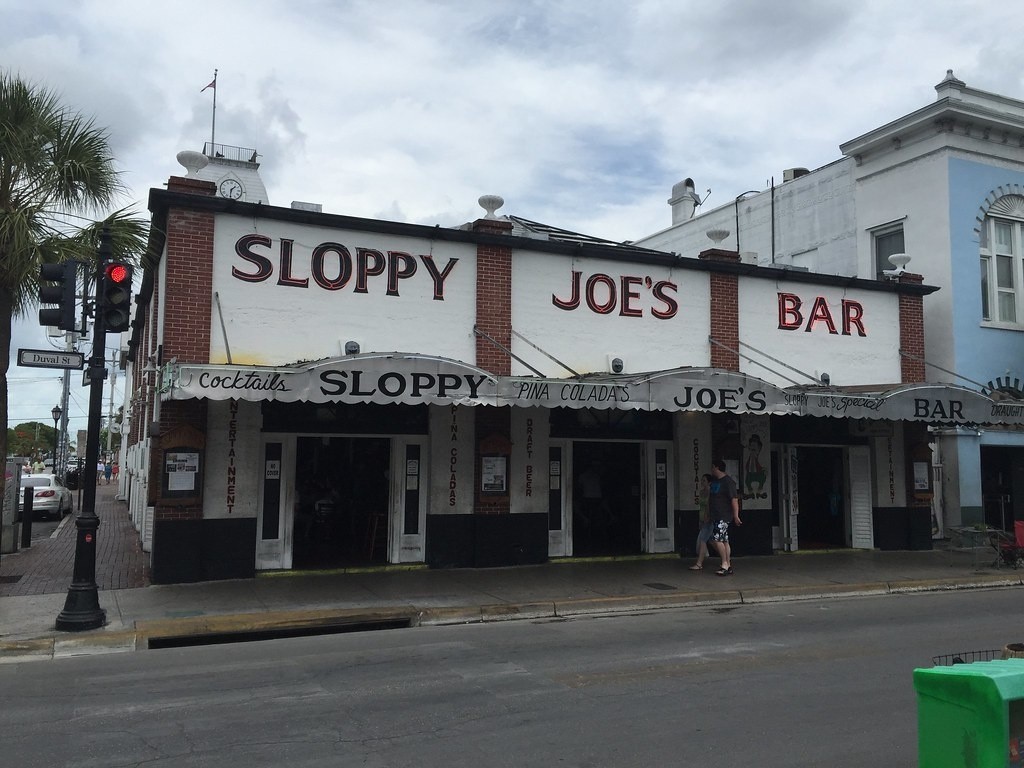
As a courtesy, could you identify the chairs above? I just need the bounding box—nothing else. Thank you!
[990,520,1024,570]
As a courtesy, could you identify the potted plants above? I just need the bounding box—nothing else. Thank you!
[971,521,990,538]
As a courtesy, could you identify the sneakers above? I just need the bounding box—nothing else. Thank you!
[714,567,733,576]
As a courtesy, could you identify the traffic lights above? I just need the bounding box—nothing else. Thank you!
[38,258,88,337]
[100,261,131,334]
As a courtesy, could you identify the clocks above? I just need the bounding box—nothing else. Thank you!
[220,180,242,200]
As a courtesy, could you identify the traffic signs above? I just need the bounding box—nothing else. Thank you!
[17,348,90,370]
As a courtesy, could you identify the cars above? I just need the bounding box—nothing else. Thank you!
[19,473,74,521]
[7,452,85,474]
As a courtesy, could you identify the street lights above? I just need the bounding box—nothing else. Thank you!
[51,404,63,475]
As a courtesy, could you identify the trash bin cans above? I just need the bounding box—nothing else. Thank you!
[912,657,1022,768]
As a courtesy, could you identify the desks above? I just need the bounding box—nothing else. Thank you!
[944,525,1005,573]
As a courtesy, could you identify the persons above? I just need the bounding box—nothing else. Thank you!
[105,462,113,485]
[97,458,105,485]
[704,459,743,576]
[32,457,46,474]
[112,461,119,484]
[688,474,714,570]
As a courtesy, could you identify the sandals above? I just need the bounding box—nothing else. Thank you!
[688,563,704,570]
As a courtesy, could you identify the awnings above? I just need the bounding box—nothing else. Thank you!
[782,380,1024,434]
[498,363,805,431]
[161,347,500,426]
